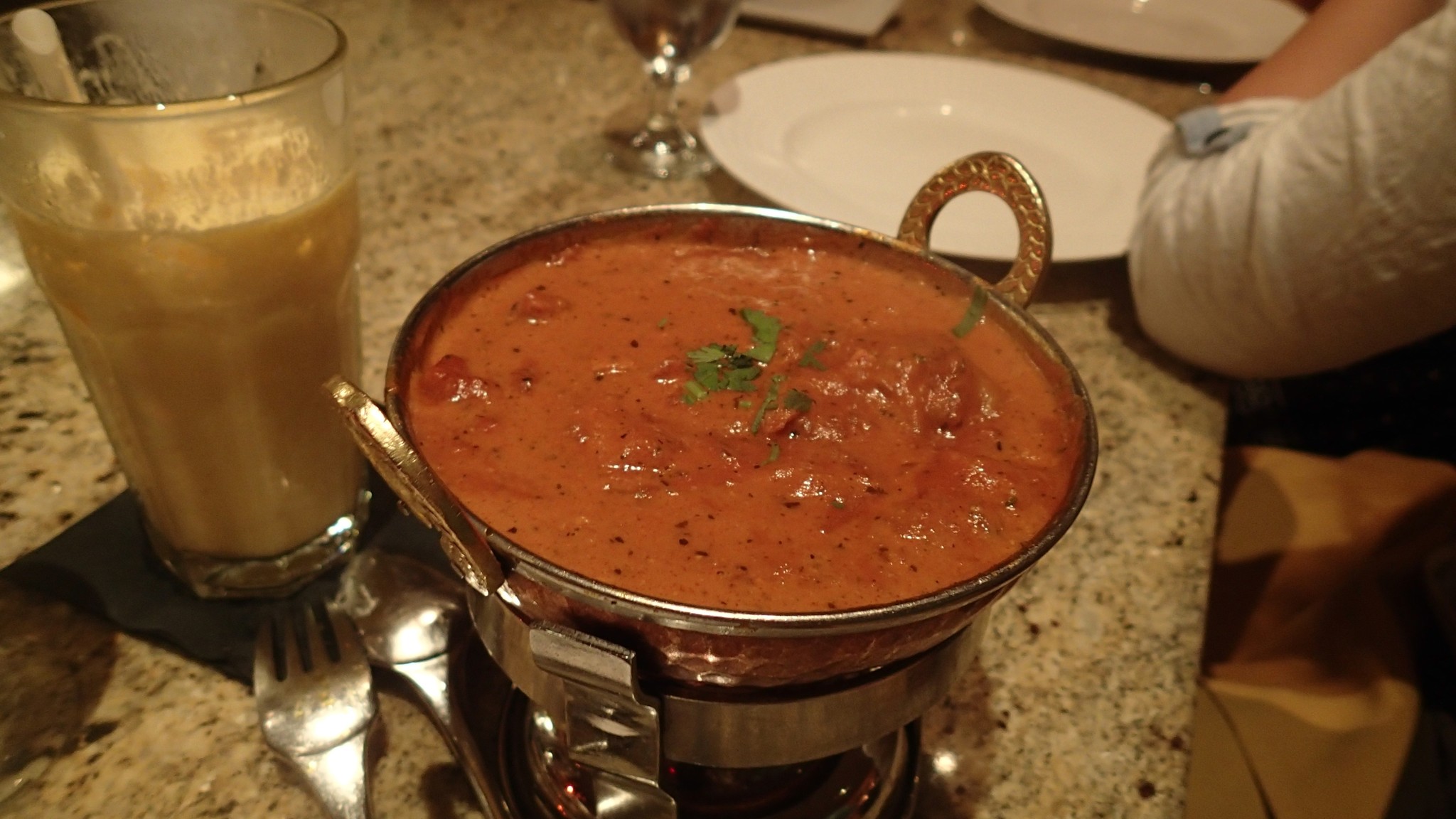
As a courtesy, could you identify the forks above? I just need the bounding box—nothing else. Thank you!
[253,598,376,819]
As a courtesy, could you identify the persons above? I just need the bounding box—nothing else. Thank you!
[1129,0,1456,377]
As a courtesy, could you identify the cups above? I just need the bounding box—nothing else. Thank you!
[0,0,363,598]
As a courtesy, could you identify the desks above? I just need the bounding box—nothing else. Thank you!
[1,0,1223,819]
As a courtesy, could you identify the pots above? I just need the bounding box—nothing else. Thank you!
[320,149,1100,698]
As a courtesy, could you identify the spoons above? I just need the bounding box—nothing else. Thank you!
[342,540,519,819]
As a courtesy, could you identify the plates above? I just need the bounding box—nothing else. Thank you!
[978,1,1308,62]
[698,52,1174,262]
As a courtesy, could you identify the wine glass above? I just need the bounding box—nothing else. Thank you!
[601,0,739,178]
